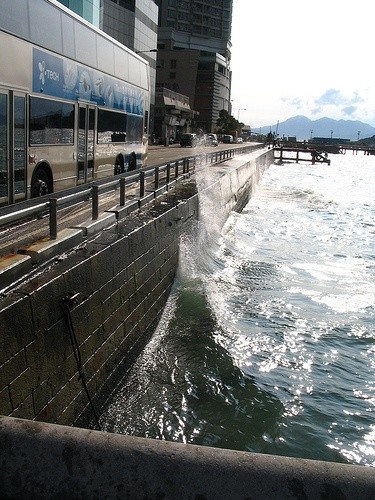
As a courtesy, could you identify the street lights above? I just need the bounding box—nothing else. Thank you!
[310,130,315,139]
[238,108,246,119]
[357,130,361,141]
[330,129,334,138]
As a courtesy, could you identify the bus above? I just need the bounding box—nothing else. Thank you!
[0,0,151,208]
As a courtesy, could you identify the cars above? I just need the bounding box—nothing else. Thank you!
[179,133,244,149]
